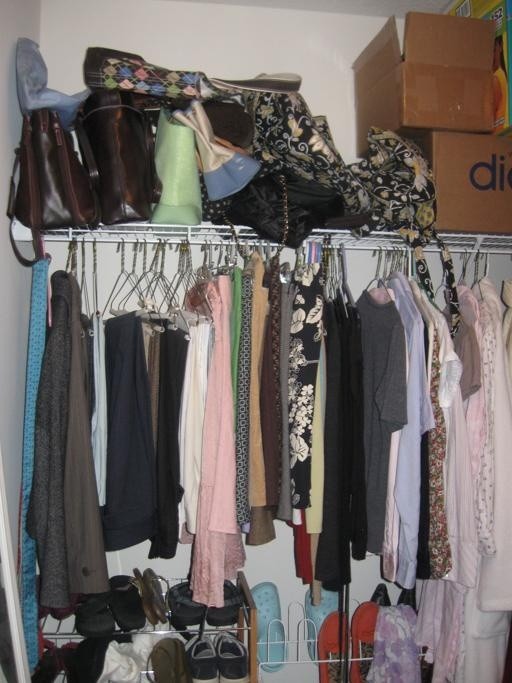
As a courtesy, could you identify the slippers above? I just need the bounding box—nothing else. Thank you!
[130,566,159,624]
[175,638,188,683]
[75,607,116,637]
[143,566,170,624]
[145,638,177,682]
[109,575,146,629]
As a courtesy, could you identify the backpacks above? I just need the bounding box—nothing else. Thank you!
[16,106,104,231]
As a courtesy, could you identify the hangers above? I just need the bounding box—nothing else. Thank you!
[365,246,490,311]
[215,238,358,308]
[38,236,215,342]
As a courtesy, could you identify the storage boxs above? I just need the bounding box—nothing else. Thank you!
[416,132,510,236]
[351,12,496,157]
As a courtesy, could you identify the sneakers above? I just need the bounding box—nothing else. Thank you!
[349,600,379,683]
[311,608,347,683]
[213,630,250,683]
[185,632,218,683]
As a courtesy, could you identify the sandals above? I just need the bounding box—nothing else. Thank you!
[168,580,205,626]
[206,579,241,626]
[303,581,341,666]
[241,582,287,672]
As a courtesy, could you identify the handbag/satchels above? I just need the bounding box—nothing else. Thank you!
[344,124,461,341]
[14,36,96,132]
[171,99,263,202]
[151,108,203,224]
[235,87,372,215]
[83,47,226,99]
[212,164,346,250]
[73,90,154,225]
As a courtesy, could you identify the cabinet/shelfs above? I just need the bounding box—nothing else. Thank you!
[27,574,258,682]
